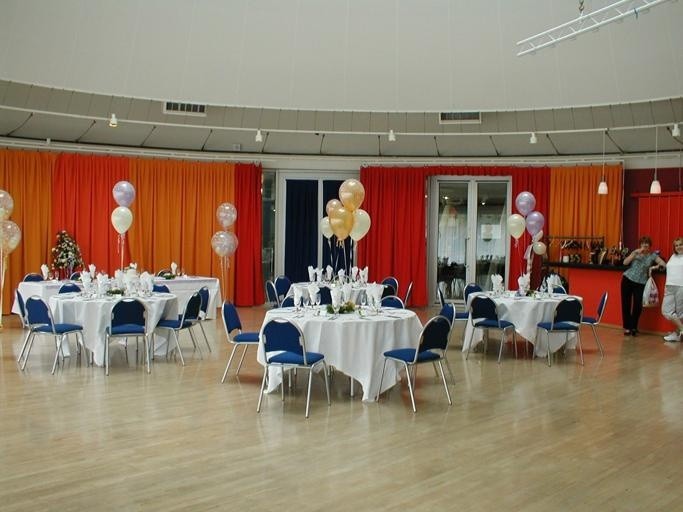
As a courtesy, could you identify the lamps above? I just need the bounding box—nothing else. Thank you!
[108,113,116,127]
[649,127,661,195]
[255,131,262,142]
[388,132,395,142]
[597,131,609,195]
[530,134,537,144]
[672,124,680,136]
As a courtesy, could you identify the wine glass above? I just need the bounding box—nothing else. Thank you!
[294,293,321,314]
[332,295,341,320]
[48,271,60,284]
[360,293,382,318]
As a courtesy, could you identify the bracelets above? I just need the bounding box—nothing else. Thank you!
[653,266,656,271]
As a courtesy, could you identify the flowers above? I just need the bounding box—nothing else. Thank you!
[49,229,85,269]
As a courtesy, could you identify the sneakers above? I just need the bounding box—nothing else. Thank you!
[624,328,639,335]
[664,332,682,342]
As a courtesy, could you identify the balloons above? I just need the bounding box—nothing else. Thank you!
[110,207,133,234]
[0,219,22,269]
[319,178,372,244]
[112,181,136,209]
[210,201,238,261]
[1,190,13,220]
[507,191,547,256]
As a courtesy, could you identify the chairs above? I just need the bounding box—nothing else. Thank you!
[437,287,469,322]
[376,315,453,413]
[257,316,331,418]
[581,289,607,358]
[220,298,261,384]
[464,282,585,367]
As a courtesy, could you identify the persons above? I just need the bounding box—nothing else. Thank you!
[662,238,683,341]
[619,235,666,336]
